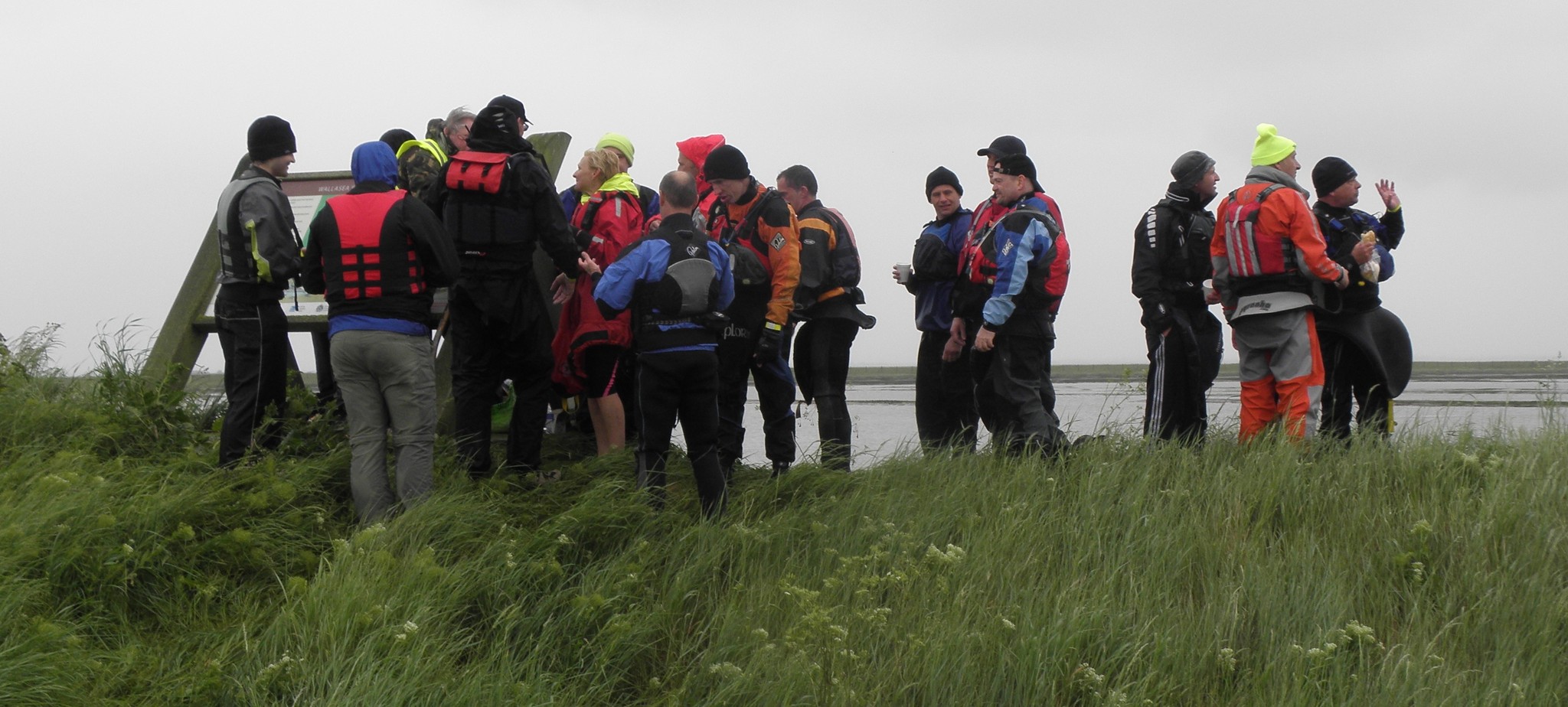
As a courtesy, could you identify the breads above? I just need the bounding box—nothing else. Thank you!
[1362,263,1374,280]
[1360,230,1376,246]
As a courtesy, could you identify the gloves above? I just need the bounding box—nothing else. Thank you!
[751,320,784,368]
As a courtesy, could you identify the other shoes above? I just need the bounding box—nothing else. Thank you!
[509,468,562,494]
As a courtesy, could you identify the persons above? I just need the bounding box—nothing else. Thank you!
[305,95,1404,527]
[216,116,302,471]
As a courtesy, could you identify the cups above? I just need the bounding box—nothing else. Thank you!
[1203,278,1214,301]
[896,262,912,283]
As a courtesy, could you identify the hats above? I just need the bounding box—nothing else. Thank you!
[1171,150,1215,188]
[704,144,750,181]
[992,154,1045,193]
[1313,156,1357,198]
[600,139,634,166]
[486,95,536,126]
[1251,124,1298,168]
[978,135,1026,160]
[247,116,297,160]
[925,166,964,203]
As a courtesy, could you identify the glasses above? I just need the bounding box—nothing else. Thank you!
[520,119,528,131]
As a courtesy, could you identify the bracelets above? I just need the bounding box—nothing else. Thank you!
[1337,267,1345,282]
[1388,202,1401,212]
